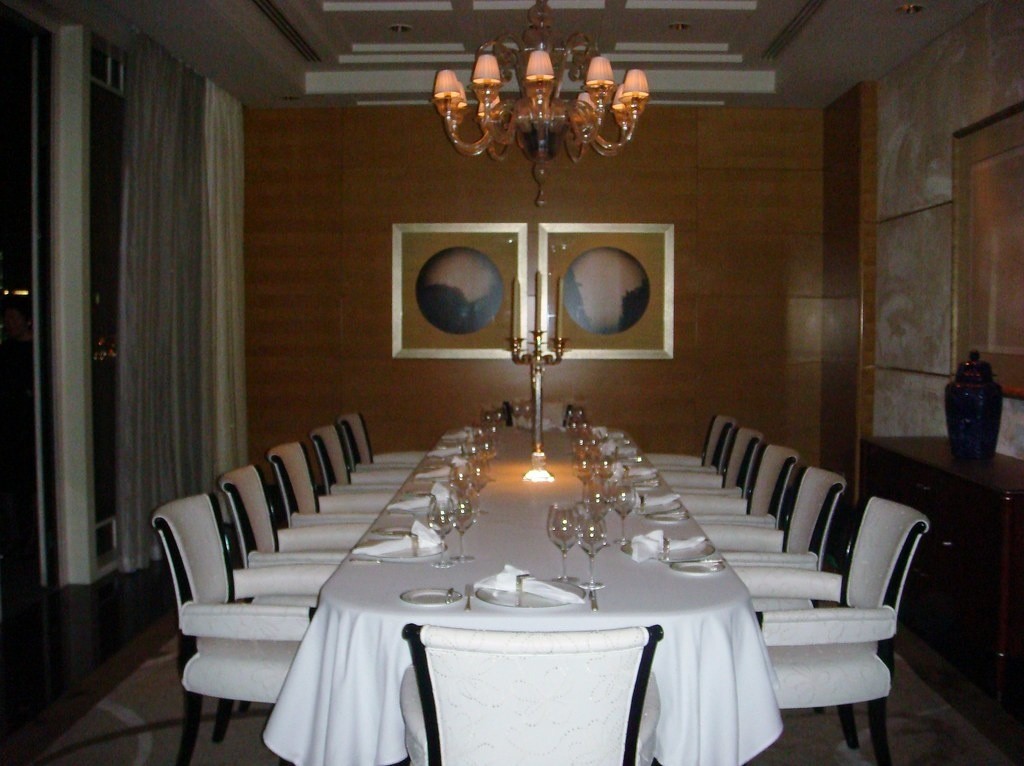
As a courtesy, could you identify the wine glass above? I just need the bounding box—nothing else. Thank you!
[425,393,531,569]
[544,408,638,593]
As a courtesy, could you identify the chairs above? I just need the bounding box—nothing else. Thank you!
[643,413,929,765]
[399,623,665,766]
[150,410,428,766]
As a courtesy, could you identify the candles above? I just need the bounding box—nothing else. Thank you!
[513,276,520,339]
[557,275,565,338]
[535,270,542,332]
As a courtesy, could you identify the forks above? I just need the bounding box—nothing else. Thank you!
[463,583,474,611]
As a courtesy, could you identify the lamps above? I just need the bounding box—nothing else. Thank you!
[430,0,650,207]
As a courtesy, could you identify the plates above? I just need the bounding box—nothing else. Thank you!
[648,534,718,561]
[475,577,588,609]
[400,586,461,605]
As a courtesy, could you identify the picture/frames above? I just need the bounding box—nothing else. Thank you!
[391,222,528,360]
[947,100,1024,397]
[538,223,674,360]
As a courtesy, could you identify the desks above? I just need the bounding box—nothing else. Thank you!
[263,429,783,766]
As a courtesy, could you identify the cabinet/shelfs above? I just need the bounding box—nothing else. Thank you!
[857,435,1024,766]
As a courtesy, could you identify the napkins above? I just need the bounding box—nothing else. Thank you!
[416,455,468,477]
[632,530,706,562]
[472,561,585,604]
[352,519,443,555]
[387,482,459,510]
[427,427,477,457]
[590,425,680,508]
